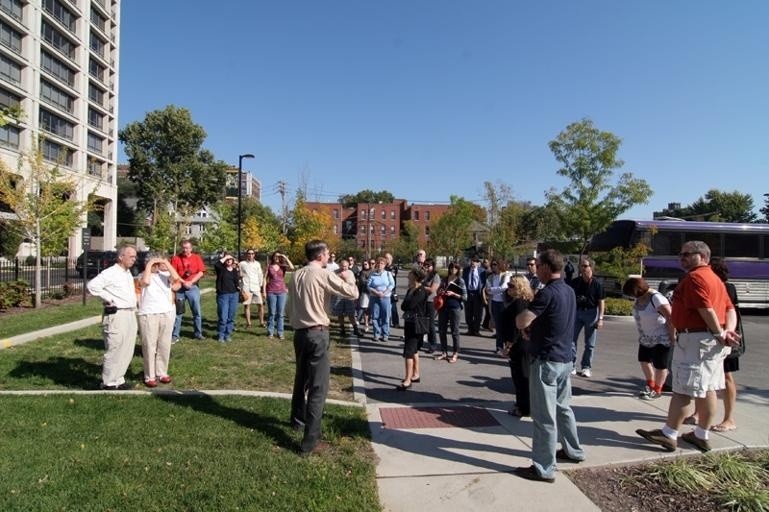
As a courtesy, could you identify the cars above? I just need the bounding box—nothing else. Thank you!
[130,252,150,276]
[165,249,271,265]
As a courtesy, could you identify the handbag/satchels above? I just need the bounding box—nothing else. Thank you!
[413,313,432,335]
[238,288,249,303]
[433,295,443,310]
[390,293,398,304]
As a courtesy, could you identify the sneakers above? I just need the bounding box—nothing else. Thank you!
[143,377,158,388]
[638,385,654,398]
[571,369,577,375]
[170,322,400,343]
[156,374,171,383]
[515,464,556,484]
[424,324,512,364]
[101,382,132,390]
[581,368,592,377]
[297,442,334,455]
[556,450,584,463]
[642,390,663,400]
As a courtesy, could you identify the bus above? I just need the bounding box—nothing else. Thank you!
[510,216,769,316]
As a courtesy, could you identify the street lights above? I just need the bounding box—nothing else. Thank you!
[238,154,255,263]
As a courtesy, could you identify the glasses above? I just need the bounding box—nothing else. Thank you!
[581,264,591,268]
[679,251,701,257]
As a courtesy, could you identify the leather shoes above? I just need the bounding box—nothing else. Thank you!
[635,428,678,452]
[682,432,712,452]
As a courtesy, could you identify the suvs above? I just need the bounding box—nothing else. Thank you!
[75,250,117,279]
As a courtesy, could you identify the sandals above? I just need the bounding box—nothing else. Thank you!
[683,416,699,424]
[397,382,413,391]
[403,377,420,383]
[509,408,530,418]
[710,422,737,432]
